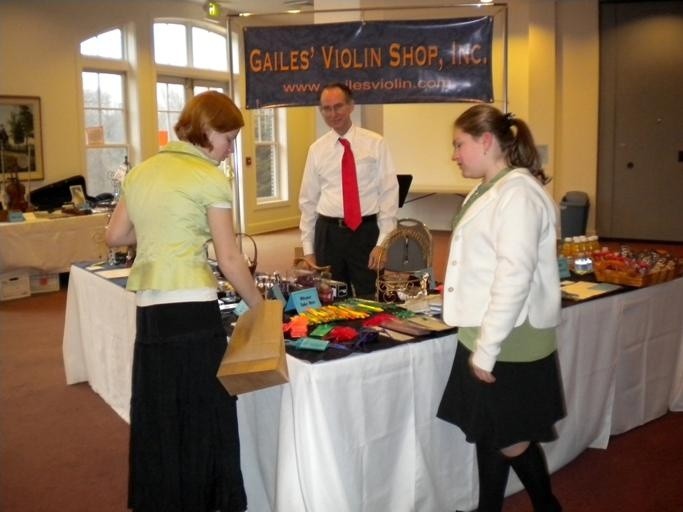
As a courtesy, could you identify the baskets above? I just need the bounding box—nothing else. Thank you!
[593,252,675,287]
[217,233,257,278]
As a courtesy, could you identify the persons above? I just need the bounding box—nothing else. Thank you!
[104,90,265,512]
[435,104,568,512]
[298,82,400,302]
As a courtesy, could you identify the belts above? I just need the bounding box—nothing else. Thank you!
[320,215,375,228]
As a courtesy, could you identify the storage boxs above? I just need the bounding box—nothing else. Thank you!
[29,272,60,294]
[0,270,32,301]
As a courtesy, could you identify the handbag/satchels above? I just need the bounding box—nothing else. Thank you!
[216,299,289,396]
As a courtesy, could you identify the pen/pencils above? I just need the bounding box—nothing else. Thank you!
[290,298,398,326]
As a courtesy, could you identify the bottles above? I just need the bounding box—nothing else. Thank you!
[561,234,600,256]
[317,286,334,305]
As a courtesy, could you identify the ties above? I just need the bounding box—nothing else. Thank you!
[338,138,363,231]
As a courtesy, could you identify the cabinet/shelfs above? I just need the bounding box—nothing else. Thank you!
[594,0,682,243]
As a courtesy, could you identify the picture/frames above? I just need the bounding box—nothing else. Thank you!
[0,95,44,183]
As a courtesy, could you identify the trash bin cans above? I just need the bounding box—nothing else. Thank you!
[560,191,590,242]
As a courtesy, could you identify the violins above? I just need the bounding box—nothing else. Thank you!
[6,157,28,212]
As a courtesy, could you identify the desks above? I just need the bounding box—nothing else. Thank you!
[0,207,128,275]
[62,261,682,512]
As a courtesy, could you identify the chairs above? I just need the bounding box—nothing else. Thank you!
[379,219,434,281]
